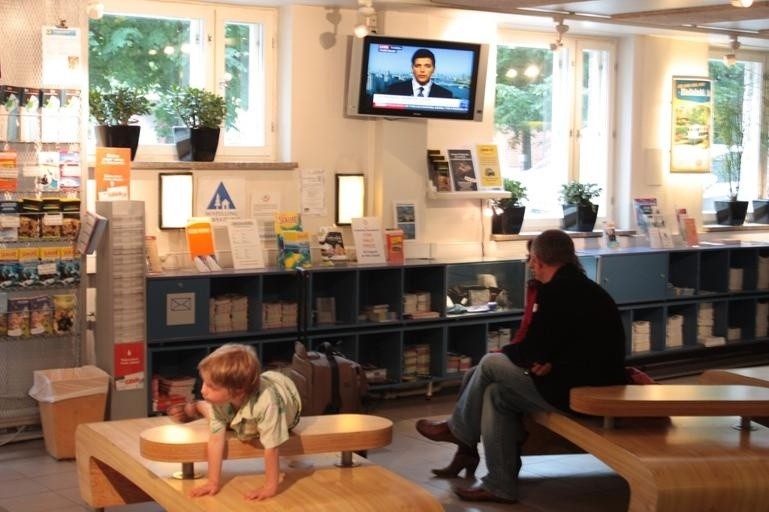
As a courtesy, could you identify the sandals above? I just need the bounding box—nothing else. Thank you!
[167,401,204,423]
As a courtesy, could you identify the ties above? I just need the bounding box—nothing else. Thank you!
[417,87,424,96]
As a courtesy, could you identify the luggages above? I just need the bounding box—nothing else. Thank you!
[290,339,369,457]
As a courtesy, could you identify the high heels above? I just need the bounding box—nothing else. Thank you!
[431,447,480,477]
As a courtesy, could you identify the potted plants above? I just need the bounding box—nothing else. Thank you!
[752,88,769,224]
[561,180,603,232]
[492,178,530,235]
[89,84,157,162]
[711,95,748,225]
[167,82,228,162]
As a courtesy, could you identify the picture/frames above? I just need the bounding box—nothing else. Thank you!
[158,171,193,229]
[669,74,714,173]
[334,173,365,226]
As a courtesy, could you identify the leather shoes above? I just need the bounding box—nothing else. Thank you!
[415,417,474,447]
[452,483,514,502]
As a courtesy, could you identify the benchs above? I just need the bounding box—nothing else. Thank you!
[530,384,769,512]
[73,413,448,511]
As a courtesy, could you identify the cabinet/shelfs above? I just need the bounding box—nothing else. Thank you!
[146,240,769,417]
[1,85,88,444]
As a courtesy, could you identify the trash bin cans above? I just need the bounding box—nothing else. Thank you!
[33,365,110,459]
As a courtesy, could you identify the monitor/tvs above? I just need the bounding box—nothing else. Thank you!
[346,36,490,122]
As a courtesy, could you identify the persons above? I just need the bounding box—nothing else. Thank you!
[167,342,302,501]
[387,50,453,98]
[431,239,545,479]
[415,229,632,503]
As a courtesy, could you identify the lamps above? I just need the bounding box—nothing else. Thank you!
[353,0,376,39]
[724,35,742,64]
[548,15,569,51]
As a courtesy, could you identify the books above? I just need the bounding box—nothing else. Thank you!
[363,285,512,377]
[209,294,337,334]
[79,212,107,254]
[631,255,769,355]
[150,375,197,419]
